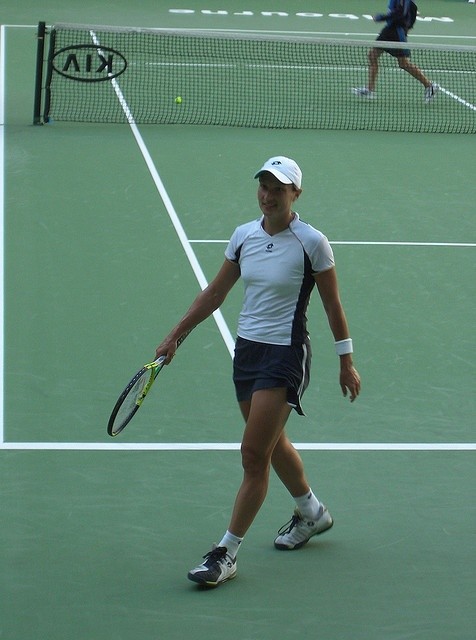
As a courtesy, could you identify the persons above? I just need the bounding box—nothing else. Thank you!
[152,155,361,587]
[352,1,441,104]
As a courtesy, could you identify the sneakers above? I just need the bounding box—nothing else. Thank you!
[187,542,237,587]
[424,82,440,104]
[274,503,334,549]
[353,86,377,101]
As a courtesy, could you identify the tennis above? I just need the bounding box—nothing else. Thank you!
[174,96,181,104]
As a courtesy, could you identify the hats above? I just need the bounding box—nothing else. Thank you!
[254,156,302,188]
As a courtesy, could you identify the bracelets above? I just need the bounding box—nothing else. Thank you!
[334,338,354,356]
